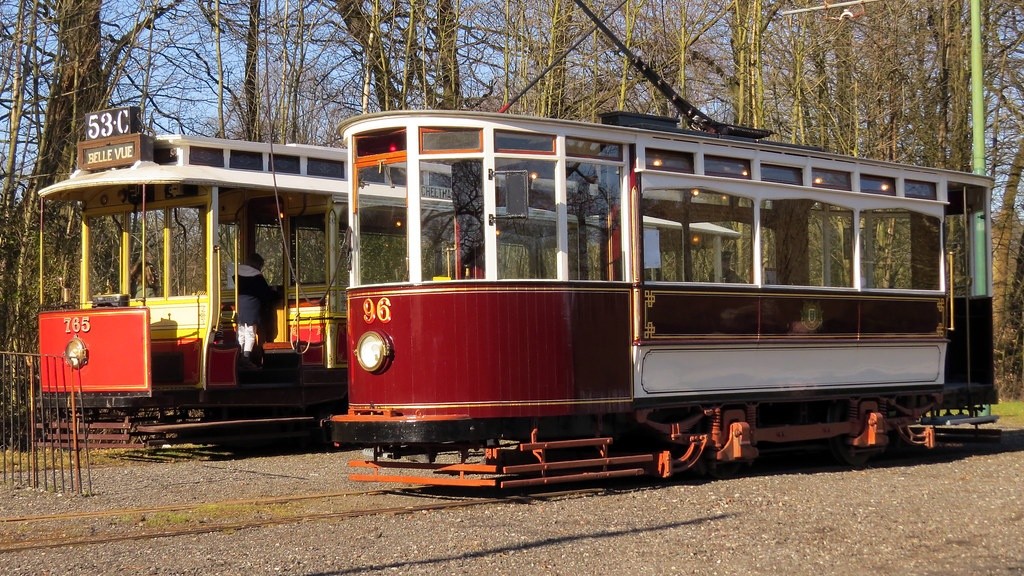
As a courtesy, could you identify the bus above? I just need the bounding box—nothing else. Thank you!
[336,108,1001,491]
[35,108,739,456]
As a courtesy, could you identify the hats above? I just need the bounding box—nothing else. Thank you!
[721,251,733,261]
[247,253,264,269]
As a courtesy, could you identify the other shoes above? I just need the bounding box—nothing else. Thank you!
[241,359,263,370]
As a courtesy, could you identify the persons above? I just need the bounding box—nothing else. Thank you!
[131,261,159,298]
[708,250,741,283]
[232,253,282,366]
